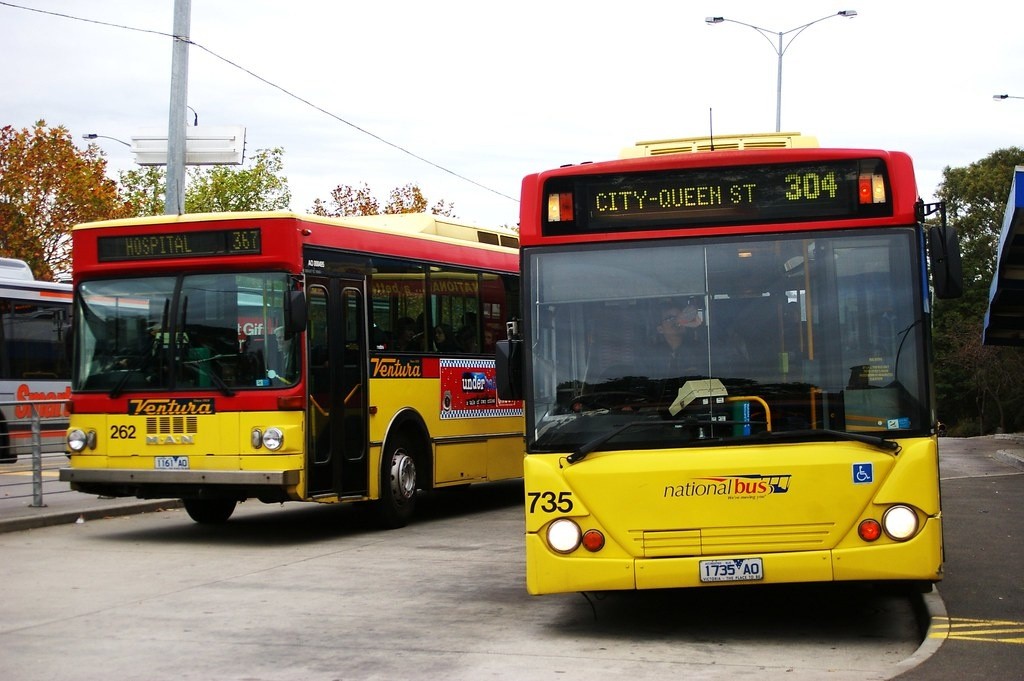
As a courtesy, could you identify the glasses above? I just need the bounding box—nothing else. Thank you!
[662,315,676,322]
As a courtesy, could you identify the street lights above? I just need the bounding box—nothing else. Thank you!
[82,133,130,148]
[705,10,857,135]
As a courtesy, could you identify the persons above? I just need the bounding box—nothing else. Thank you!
[394,313,496,354]
[640,301,720,378]
[274,325,301,385]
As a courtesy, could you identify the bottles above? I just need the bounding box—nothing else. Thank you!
[676,305,699,328]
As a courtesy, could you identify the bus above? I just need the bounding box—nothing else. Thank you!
[59,210,522,525]
[496,133,966,597]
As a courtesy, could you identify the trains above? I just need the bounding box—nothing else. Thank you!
[0,258,283,464]
